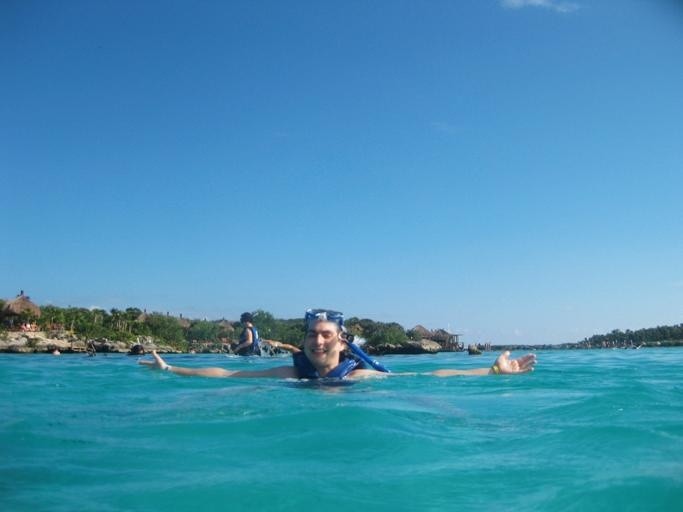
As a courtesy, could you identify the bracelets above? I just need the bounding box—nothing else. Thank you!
[492,365,500,375]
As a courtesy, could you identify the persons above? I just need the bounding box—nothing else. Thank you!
[20,320,38,331]
[131,345,144,354]
[263,339,301,353]
[234,312,260,356]
[139,308,538,378]
